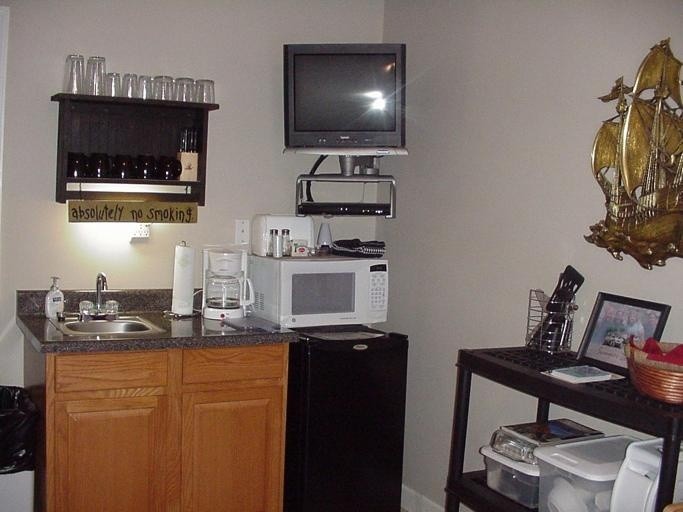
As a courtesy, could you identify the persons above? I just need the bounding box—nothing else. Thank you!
[591,305,658,348]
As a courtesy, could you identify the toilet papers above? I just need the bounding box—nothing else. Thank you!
[170,245,196,315]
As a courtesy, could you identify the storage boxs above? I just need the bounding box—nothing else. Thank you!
[477,445,540,509]
[532,434,645,511]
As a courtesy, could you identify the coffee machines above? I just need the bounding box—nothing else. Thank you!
[198,245,257,321]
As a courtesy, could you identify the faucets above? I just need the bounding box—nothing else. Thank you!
[96,272,108,313]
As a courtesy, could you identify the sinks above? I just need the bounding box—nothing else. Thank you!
[50,315,168,337]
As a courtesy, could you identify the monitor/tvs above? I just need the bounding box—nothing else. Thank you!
[282,42,407,149]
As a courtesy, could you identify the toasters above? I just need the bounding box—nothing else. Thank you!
[251,213,316,259]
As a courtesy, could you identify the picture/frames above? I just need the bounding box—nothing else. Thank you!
[573,291,671,379]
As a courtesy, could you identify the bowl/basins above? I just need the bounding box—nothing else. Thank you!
[546,475,587,512]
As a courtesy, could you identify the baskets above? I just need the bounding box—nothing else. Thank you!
[622,332,683,406]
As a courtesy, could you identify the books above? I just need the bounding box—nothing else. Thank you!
[503,418,605,447]
[551,366,611,382]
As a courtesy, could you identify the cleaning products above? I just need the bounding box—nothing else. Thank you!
[44,276,64,319]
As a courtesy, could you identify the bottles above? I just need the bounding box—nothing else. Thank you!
[317,223,333,250]
[269,228,292,258]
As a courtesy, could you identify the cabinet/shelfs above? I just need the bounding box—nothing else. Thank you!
[441,345,682,512]
[281,326,410,512]
[21,335,289,512]
[49,92,220,207]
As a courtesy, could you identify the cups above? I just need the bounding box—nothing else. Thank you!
[63,54,215,103]
[339,155,356,177]
[68,127,197,179]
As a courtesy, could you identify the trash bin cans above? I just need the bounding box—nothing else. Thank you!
[0,386,39,512]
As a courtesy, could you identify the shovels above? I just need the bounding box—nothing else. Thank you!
[527,265,584,351]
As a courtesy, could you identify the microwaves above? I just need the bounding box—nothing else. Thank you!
[245,253,391,328]
